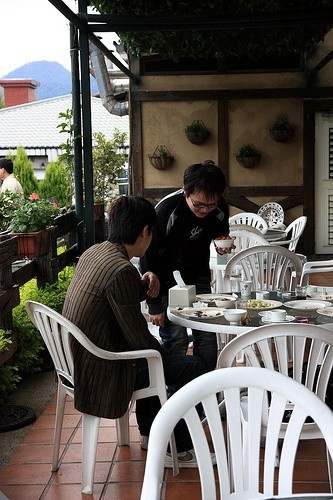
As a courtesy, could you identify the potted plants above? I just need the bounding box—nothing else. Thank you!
[94,187,104,220]
[149,146,175,170]
[12,201,59,259]
[236,144,262,169]
[186,119,212,145]
[54,189,77,238]
[271,114,296,144]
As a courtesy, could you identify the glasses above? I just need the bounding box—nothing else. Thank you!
[188,192,218,208]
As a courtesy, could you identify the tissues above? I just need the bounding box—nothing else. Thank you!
[167,269,196,309]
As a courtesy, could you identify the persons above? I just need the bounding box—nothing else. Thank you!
[141,164,235,371]
[0,158,22,194]
[61,196,218,468]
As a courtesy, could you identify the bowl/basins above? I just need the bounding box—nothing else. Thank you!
[214,240,234,248]
[214,297,236,309]
[223,308,247,324]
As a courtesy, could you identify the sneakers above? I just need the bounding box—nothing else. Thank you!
[164,447,217,468]
[140,435,148,450]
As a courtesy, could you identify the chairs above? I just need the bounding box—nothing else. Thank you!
[26,200,333,500]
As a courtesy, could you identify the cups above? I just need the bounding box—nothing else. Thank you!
[266,309,286,321]
[236,301,247,323]
[306,286,317,295]
[239,280,252,296]
[256,290,270,300]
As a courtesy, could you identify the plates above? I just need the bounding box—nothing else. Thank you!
[235,299,282,309]
[179,307,227,320]
[258,311,295,323]
[196,293,237,302]
[283,295,333,317]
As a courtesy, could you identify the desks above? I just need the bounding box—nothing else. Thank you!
[209,251,307,294]
[167,290,333,335]
[262,230,286,239]
